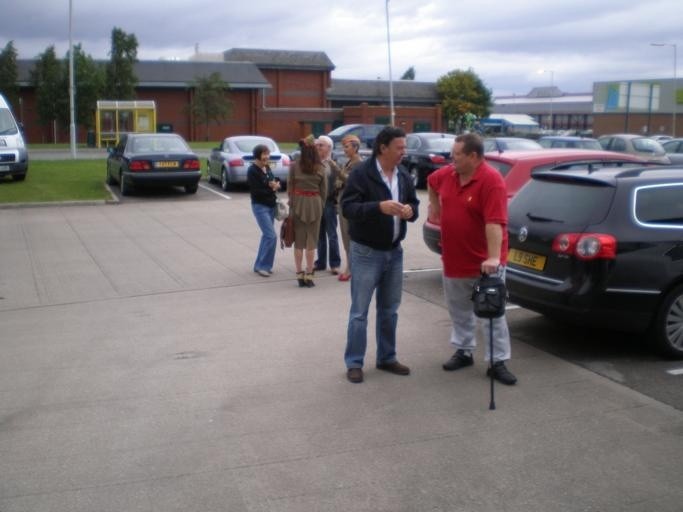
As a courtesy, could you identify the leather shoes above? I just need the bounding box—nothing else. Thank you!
[376,361,409,375]
[331,267,338,274]
[346,368,363,382]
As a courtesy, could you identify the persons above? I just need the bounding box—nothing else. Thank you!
[339,126,421,382]
[246,143,280,278]
[426,131,520,386]
[281,133,364,286]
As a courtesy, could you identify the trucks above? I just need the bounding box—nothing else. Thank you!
[329,120,390,152]
[0,94,29,181]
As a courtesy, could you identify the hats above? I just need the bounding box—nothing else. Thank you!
[341,135,359,145]
[303,134,314,146]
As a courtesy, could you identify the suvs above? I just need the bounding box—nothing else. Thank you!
[498,162,683,359]
[421,146,652,256]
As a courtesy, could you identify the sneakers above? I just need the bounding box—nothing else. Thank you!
[442,349,472,370]
[486,361,516,384]
[253,267,268,276]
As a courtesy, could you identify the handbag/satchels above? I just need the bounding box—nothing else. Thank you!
[470,272,506,318]
[274,189,288,220]
[279,207,294,249]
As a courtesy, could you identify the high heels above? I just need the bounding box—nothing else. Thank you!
[338,272,351,281]
[295,271,305,287]
[305,273,315,287]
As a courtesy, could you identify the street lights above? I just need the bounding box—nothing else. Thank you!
[650,40,676,134]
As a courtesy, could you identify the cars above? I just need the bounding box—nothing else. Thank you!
[543,132,682,164]
[104,127,292,197]
[397,123,543,186]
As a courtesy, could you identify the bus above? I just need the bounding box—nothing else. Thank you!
[488,114,543,143]
